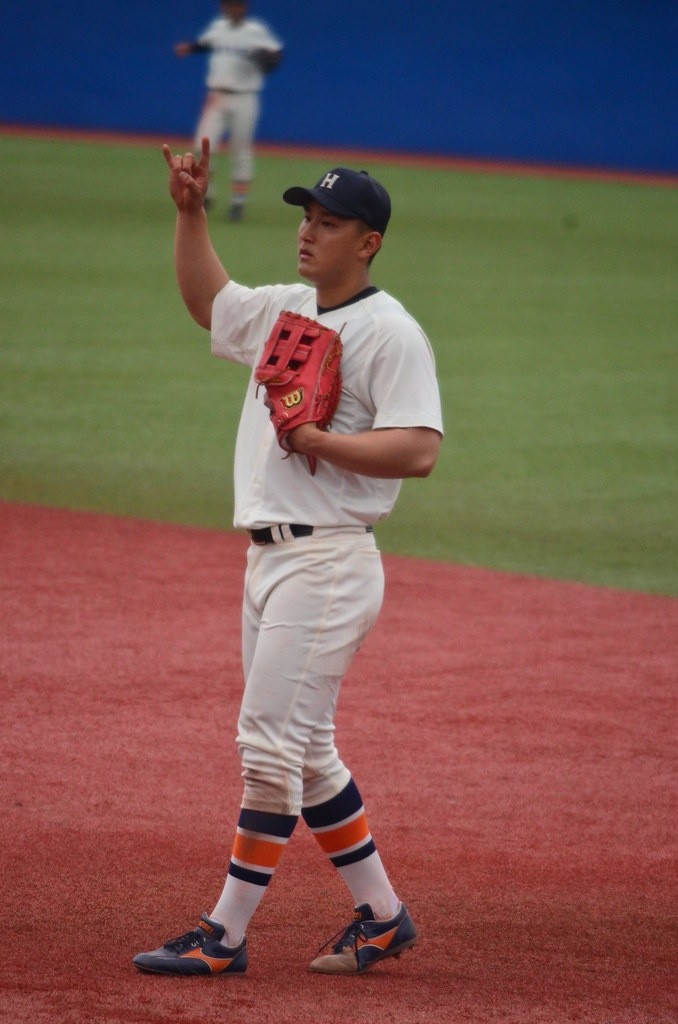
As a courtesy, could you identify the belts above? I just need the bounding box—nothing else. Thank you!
[247,521,374,546]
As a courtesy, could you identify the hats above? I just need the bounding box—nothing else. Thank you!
[282,167,390,238]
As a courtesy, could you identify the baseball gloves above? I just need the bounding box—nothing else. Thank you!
[250,308,349,480]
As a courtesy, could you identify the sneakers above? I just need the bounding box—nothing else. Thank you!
[308,902,417,973]
[133,912,248,976]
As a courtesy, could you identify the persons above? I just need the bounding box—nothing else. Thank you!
[133,137,444,976]
[174,0,282,223]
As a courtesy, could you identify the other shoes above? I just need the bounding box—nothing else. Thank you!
[229,203,243,223]
